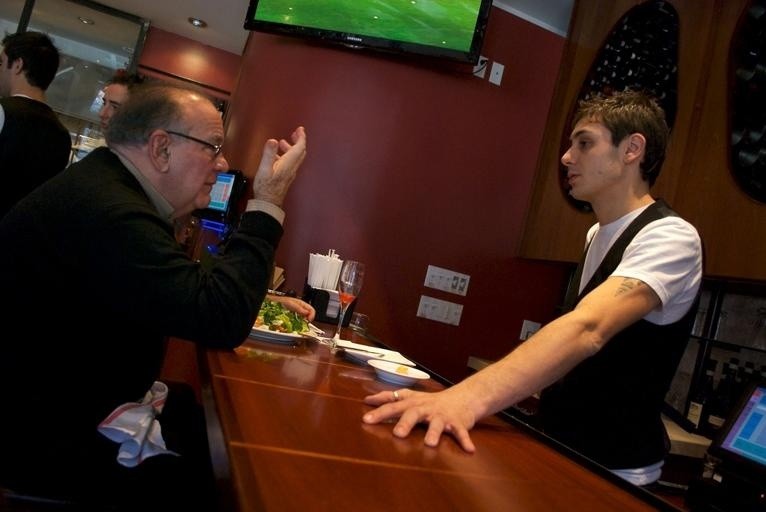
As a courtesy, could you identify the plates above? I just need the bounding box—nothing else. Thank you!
[252,321,316,339]
[337,340,416,367]
[367,359,430,387]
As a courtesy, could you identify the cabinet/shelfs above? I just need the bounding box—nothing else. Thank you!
[661,274,766,459]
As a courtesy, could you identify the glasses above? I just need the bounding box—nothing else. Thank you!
[168,131,222,156]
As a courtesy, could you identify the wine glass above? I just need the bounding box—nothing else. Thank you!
[322,260,365,345]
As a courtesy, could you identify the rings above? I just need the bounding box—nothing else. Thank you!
[393,389,400,402]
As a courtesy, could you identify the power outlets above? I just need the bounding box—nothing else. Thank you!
[472,56,489,78]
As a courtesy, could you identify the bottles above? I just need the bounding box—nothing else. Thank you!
[684,357,766,440]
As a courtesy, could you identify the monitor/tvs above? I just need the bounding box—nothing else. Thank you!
[706,384,766,478]
[196,170,249,226]
[243,1,492,66]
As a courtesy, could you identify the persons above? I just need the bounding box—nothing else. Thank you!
[1,78,308,509]
[0,29,73,222]
[98,67,148,144]
[361,85,707,493]
